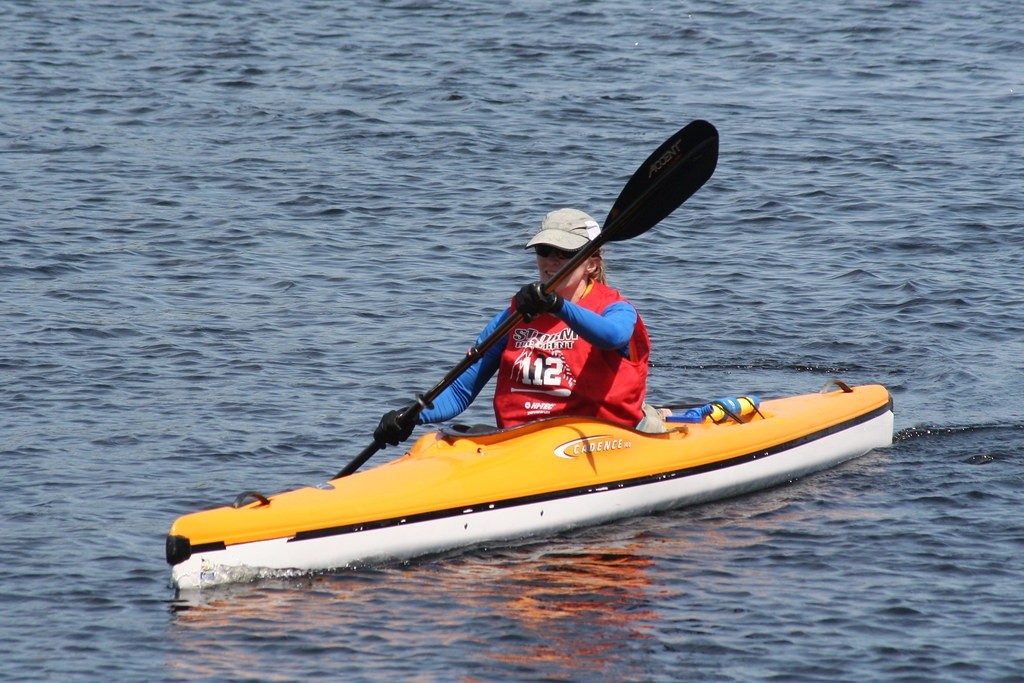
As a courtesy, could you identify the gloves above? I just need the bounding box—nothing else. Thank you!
[515,281,564,324]
[373,407,420,449]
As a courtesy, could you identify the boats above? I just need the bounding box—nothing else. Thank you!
[165,376,893,589]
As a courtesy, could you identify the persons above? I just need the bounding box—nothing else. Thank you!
[374,206,651,450]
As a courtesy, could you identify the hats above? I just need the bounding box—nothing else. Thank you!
[525,208,602,251]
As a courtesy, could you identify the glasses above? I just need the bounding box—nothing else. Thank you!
[534,245,581,259]
